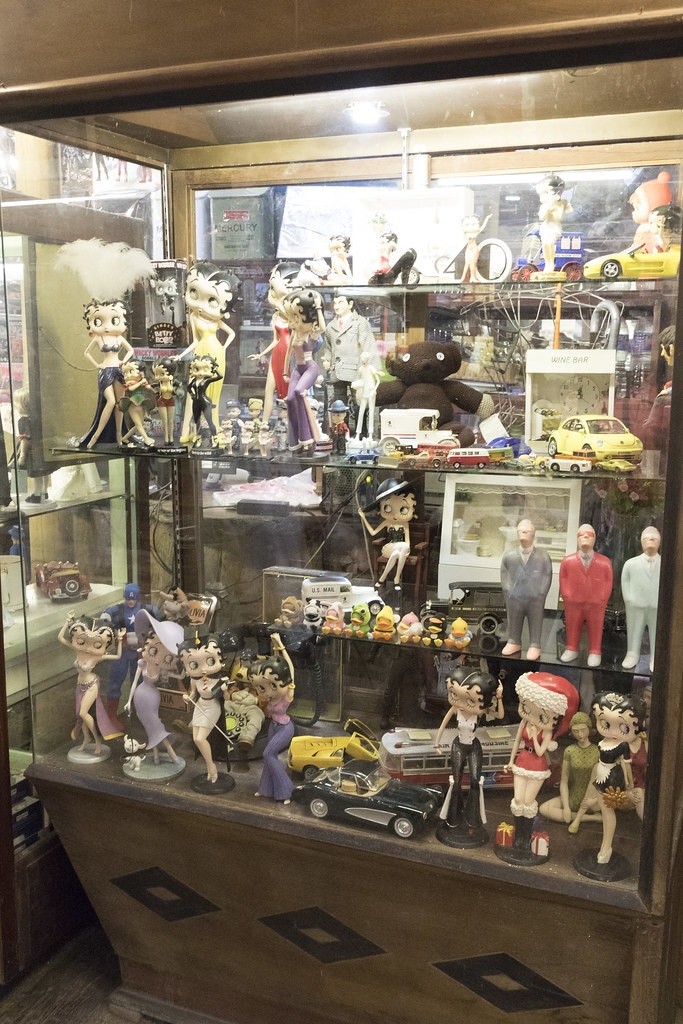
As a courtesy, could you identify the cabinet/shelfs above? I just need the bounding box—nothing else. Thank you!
[43,280,678,904]
[0,190,145,642]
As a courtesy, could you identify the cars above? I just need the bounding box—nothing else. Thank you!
[290,758,447,841]
[35,562,94,603]
[546,414,645,464]
[346,444,638,475]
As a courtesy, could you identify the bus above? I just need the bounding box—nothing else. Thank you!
[372,724,563,794]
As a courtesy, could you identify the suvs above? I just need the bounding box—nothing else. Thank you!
[420,580,509,635]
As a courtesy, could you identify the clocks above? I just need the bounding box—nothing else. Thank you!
[560,378,600,416]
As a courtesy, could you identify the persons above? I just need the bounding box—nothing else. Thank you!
[3,237,676,512]
[320,169,683,284]
[8,524,31,586]
[56,581,652,881]
[273,476,661,673]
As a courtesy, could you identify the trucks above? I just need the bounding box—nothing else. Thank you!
[378,408,462,456]
[302,576,387,620]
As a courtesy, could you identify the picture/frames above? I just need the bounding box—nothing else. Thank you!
[439,472,583,574]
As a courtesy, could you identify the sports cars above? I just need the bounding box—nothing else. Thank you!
[583,242,679,283]
[288,718,381,782]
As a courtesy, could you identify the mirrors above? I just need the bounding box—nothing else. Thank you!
[475,240,512,282]
